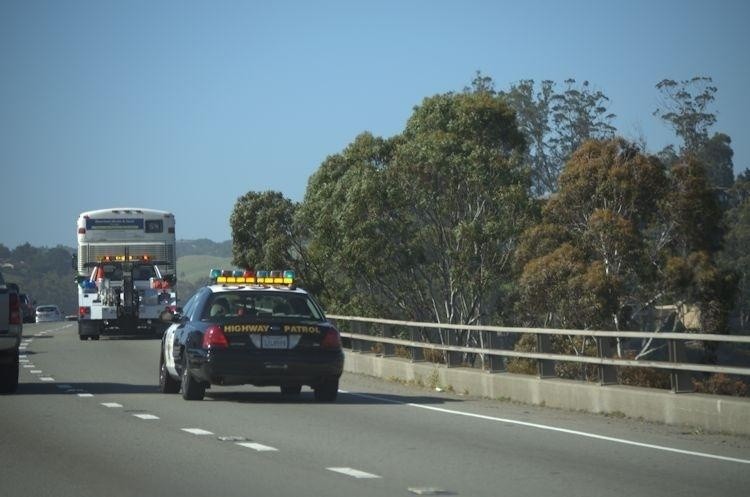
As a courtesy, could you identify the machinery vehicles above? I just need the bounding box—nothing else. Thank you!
[75,248,177,343]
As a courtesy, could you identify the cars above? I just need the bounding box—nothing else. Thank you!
[158,263,345,404]
[0,266,63,389]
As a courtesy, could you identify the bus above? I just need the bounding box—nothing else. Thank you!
[74,206,177,302]
